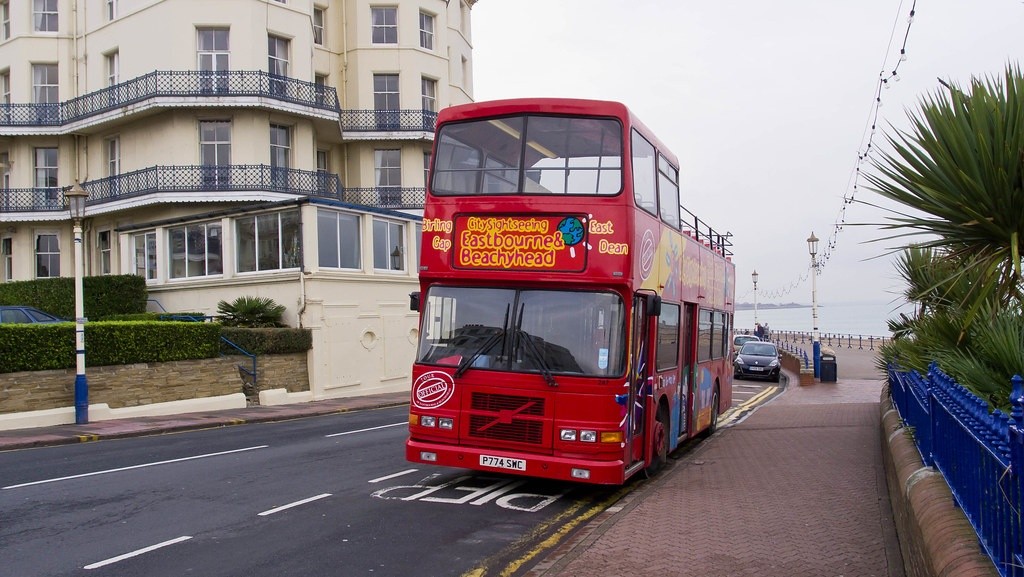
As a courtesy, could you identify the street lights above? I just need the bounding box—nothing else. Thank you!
[806,230,820,379]
[751,269,759,336]
[64,177,89,426]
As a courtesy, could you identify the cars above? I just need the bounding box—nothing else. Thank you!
[734,341,783,383]
[0,306,67,324]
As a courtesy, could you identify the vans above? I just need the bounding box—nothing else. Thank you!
[732,334,760,361]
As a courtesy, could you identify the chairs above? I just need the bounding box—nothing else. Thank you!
[635,193,731,263]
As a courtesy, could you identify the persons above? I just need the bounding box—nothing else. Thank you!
[758,323,769,339]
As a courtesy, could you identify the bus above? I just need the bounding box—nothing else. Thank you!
[402,97,737,487]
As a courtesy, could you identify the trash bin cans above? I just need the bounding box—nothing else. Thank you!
[820,353,837,382]
